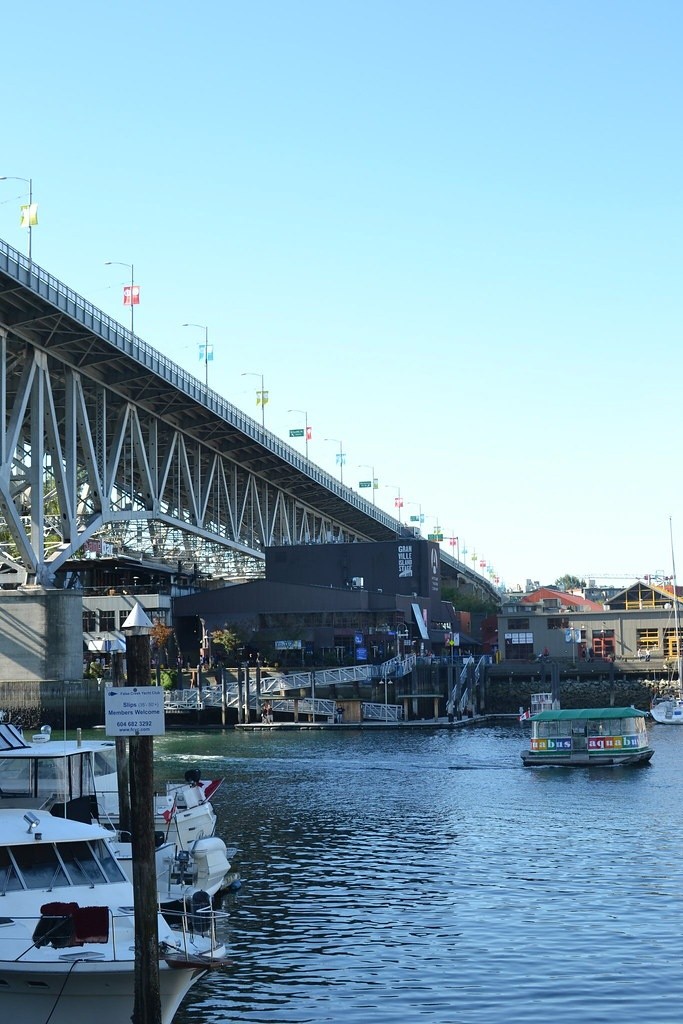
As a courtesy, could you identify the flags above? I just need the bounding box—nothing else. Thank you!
[518,711,529,721]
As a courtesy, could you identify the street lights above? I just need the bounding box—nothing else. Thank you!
[358,465,374,505]
[407,502,454,557]
[241,372,264,427]
[182,324,208,385]
[324,439,343,483]
[386,485,400,521]
[288,410,308,459]
[0,176,32,271]
[105,262,134,332]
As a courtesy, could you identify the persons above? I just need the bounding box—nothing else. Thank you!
[186,655,192,669]
[543,646,550,662]
[645,648,651,662]
[638,648,643,661]
[247,652,262,667]
[568,605,576,612]
[261,705,273,724]
[199,655,205,666]
[336,705,346,723]
[581,646,594,662]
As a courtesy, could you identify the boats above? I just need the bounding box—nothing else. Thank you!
[520,707,656,767]
[0,711,242,1024]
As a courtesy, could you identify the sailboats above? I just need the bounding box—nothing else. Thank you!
[649,516,683,725]
